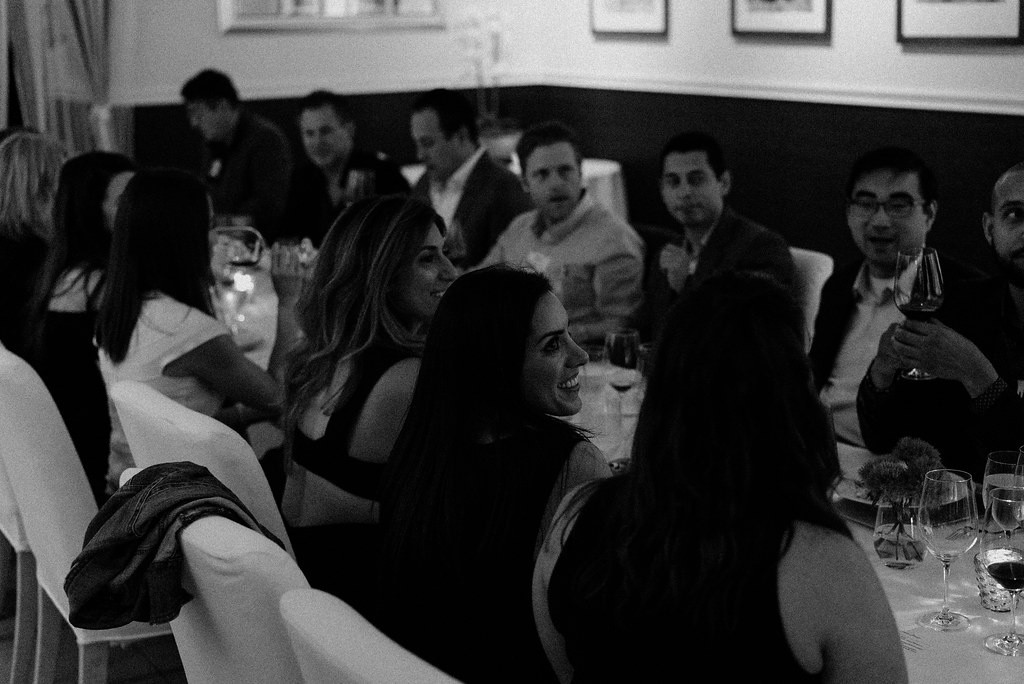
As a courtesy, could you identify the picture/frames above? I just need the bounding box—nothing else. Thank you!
[587,0,669,36]
[895,0,1024,46]
[730,0,833,42]
[213,0,449,31]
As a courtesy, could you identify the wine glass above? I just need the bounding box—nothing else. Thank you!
[579,331,654,450]
[980,446,1024,656]
[893,245,945,381]
[211,227,318,269]
[919,469,978,631]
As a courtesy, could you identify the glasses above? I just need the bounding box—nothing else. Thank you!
[848,190,926,218]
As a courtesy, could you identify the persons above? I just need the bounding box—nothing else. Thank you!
[0,69,1023,684]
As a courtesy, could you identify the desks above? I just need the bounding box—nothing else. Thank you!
[231,269,1024,684]
[402,156,626,217]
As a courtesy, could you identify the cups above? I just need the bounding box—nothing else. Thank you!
[976,549,1023,612]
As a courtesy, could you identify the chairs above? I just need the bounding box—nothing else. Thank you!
[786,248,834,356]
[0,341,463,684]
